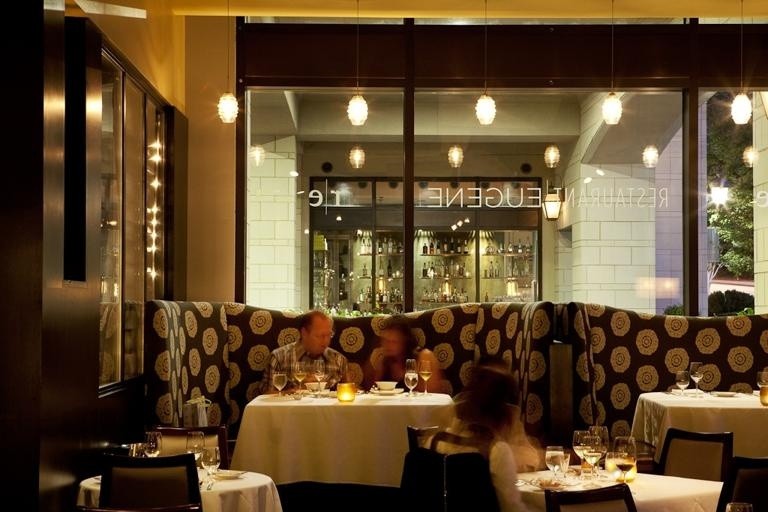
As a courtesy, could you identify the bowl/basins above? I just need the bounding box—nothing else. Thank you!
[303,381,327,390]
[374,382,399,390]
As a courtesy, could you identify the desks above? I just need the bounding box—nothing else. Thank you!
[79,468,273,512]
[243,393,457,483]
[516,466,723,510]
[638,391,766,458]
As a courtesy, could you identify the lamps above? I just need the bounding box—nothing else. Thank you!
[215,0,240,125]
[598,0,624,126]
[350,146,364,170]
[544,146,560,169]
[474,1,498,126]
[251,145,265,170]
[743,145,753,167]
[343,0,370,127]
[537,171,566,222]
[643,145,657,169]
[448,146,464,168]
[728,0,754,128]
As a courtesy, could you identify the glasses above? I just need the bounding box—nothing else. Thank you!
[314,331,334,340]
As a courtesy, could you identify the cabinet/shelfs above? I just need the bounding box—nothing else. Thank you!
[353,244,533,313]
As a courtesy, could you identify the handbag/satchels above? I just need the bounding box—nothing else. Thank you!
[182,395,211,428]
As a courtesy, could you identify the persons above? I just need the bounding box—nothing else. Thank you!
[258,309,351,395]
[448,354,541,471]
[423,369,529,511]
[364,314,445,394]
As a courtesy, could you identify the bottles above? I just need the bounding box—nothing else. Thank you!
[421,236,469,305]
[360,236,404,254]
[484,237,531,281]
[362,257,392,278]
[359,286,401,303]
[485,292,489,302]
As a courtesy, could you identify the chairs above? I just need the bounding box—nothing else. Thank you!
[76,384,767,511]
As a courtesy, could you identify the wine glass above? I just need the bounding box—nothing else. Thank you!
[201,446,221,488]
[311,359,326,393]
[674,370,691,396]
[293,361,308,394]
[272,374,287,398]
[403,374,419,397]
[690,360,704,398]
[545,425,637,489]
[419,360,432,396]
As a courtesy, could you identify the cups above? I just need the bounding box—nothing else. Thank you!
[128,443,145,459]
[144,430,162,457]
[759,387,768,407]
[336,383,355,402]
[405,357,416,372]
[755,370,768,387]
[185,431,205,463]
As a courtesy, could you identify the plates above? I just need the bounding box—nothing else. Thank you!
[216,469,247,479]
[369,388,406,396]
[709,391,737,396]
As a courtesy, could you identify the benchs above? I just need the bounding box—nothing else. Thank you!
[562,302,767,444]
[143,299,561,444]
